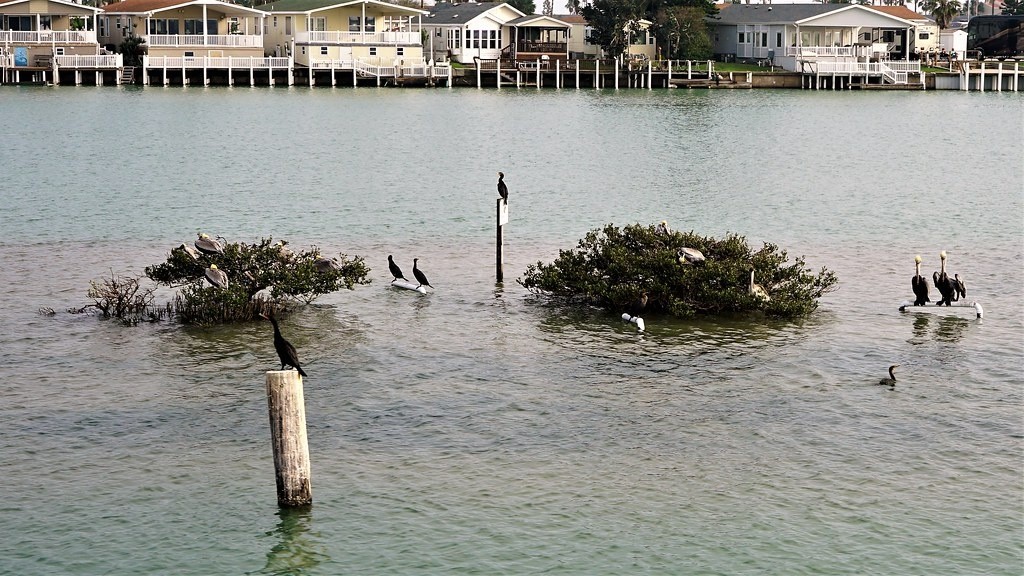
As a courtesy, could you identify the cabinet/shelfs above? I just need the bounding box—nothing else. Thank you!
[874,51,890,62]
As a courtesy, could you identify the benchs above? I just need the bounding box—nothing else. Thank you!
[33,54,53,68]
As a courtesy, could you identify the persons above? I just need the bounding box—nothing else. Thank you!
[940,48,956,61]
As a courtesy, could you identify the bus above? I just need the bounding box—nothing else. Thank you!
[967,15,1024,61]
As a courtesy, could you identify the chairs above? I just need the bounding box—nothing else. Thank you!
[542,55,550,70]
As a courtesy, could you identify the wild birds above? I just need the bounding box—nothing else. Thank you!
[413,258,434,289]
[912,255,931,306]
[496,171,509,205]
[194,231,221,256]
[747,266,774,307]
[388,255,409,283]
[266,313,308,377]
[933,251,966,307]
[881,364,901,385]
[204,264,229,291]
[656,221,672,236]
[676,247,706,269]
[171,243,200,267]
